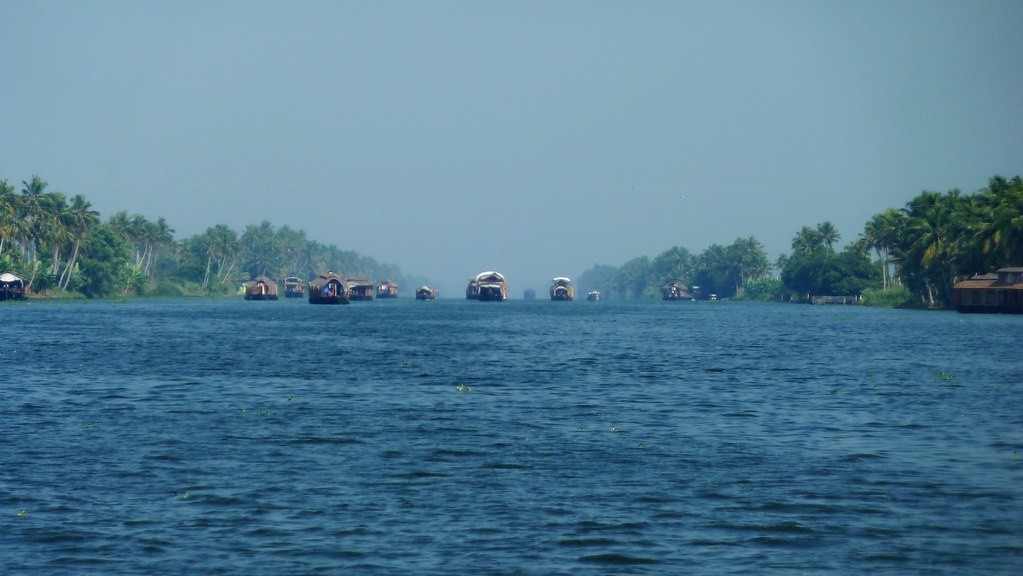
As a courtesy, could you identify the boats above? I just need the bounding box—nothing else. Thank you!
[662,279,693,300]
[522,288,536,300]
[476,271,507,301]
[550,276,573,300]
[375,279,399,298]
[245,274,278,300]
[416,284,435,299]
[466,277,478,299]
[951,267,1023,313]
[285,276,306,298]
[587,290,601,301]
[1,272,25,301]
[307,271,349,305]
[709,293,717,300]
[346,277,374,300]
